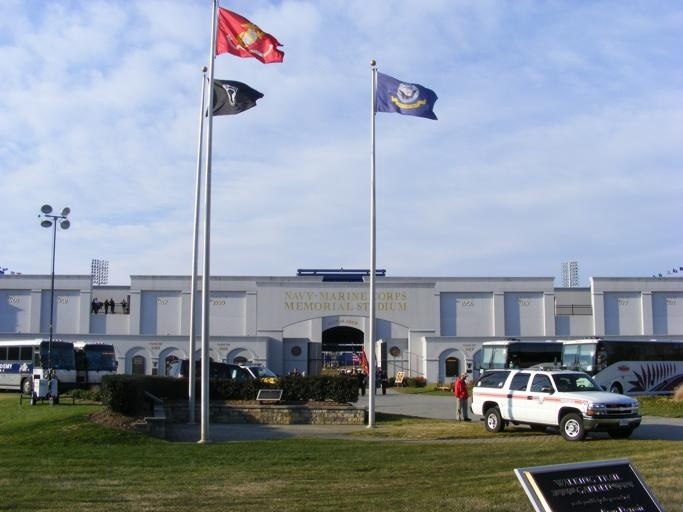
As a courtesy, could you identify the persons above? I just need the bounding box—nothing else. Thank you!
[454,373,471,422]
[91,297,127,314]
[340,369,388,395]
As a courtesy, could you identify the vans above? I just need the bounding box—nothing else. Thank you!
[166,359,249,379]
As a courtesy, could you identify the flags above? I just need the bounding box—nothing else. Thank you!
[206,77,264,117]
[353,348,369,374]
[374,69,439,120]
[215,6,284,64]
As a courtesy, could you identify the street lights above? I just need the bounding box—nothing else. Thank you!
[37,204,70,378]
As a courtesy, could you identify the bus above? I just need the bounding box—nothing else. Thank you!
[72,342,118,384]
[0,339,76,395]
[467,338,563,373]
[553,338,682,396]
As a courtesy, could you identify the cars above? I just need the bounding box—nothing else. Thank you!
[239,366,278,379]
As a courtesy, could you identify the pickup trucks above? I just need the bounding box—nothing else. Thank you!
[471,367,641,441]
